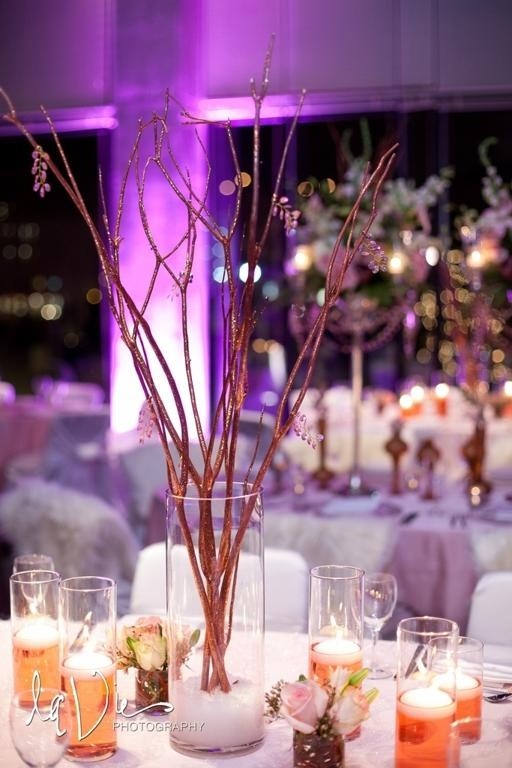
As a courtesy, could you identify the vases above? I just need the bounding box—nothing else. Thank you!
[135,670,167,714]
[291,728,344,767]
[164,479,267,754]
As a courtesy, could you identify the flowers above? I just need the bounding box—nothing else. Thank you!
[276,670,378,739]
[111,618,201,679]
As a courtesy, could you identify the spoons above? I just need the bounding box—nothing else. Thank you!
[483,692,511,704]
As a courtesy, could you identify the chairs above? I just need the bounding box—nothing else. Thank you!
[0,477,313,640]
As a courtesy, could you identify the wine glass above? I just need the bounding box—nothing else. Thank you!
[9,686,73,768]
[13,553,55,614]
[353,572,398,680]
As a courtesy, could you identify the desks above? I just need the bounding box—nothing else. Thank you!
[262,471,512,638]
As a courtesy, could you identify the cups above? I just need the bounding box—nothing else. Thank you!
[8,569,63,708]
[393,614,461,768]
[426,635,484,745]
[57,574,120,765]
[306,565,366,742]
